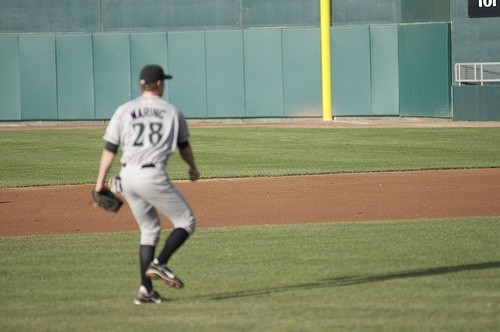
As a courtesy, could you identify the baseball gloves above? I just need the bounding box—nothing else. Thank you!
[91,187,123,213]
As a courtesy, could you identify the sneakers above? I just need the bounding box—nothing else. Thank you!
[134,291,170,305]
[145,262,184,290]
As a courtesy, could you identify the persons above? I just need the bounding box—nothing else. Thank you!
[91,65,200,304]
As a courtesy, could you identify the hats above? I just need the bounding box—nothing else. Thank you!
[139,65,172,86]
